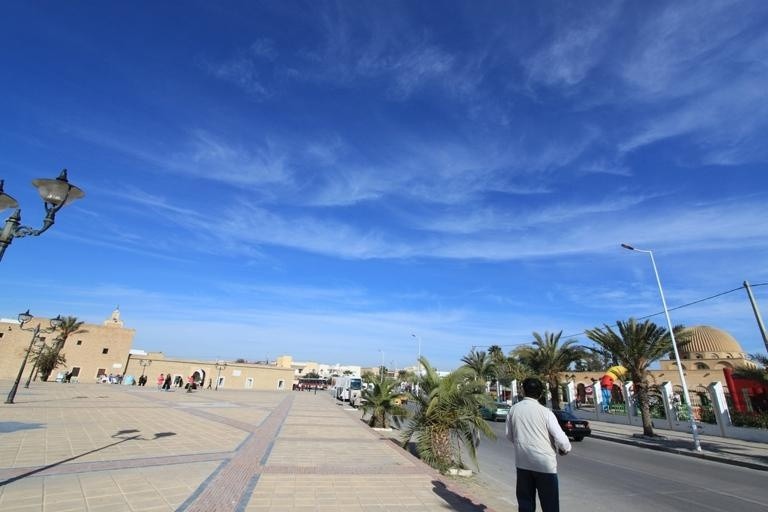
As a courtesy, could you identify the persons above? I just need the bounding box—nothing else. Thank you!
[504,376,572,511]
[156,373,195,393]
[95,371,149,388]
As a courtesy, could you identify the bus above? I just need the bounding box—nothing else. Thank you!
[335,376,362,401]
[297,377,328,389]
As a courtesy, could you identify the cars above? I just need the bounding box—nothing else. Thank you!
[480,401,513,423]
[550,408,592,443]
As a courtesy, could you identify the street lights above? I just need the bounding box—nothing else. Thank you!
[5,307,67,404]
[412,334,419,397]
[214,362,229,390]
[139,358,152,375]
[378,348,384,384]
[0,168,88,264]
[23,336,64,388]
[619,242,703,451]
